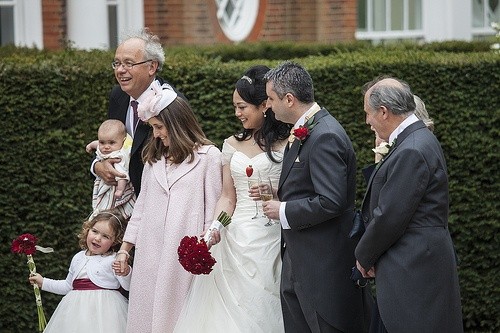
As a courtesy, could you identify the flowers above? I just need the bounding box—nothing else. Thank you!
[177,210,232,275]
[371,142,391,158]
[12,233,53,330]
[292,116,320,141]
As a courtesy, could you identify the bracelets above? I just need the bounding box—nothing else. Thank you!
[116,250,130,260]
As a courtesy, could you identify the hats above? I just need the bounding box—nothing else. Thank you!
[135,80,179,123]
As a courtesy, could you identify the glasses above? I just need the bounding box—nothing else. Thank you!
[111,58,153,70]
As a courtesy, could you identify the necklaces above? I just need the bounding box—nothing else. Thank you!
[251,139,257,145]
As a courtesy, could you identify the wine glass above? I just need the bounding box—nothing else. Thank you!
[247,179,259,219]
[258,177,280,227]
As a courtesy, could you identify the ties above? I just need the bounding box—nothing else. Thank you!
[130,100,139,133]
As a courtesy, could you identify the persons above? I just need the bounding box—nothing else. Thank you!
[173,65,294,333]
[362,94,434,184]
[113,80,223,333]
[106,28,189,300]
[355,76,464,333]
[29,209,133,333]
[262,61,376,333]
[85,119,136,223]
